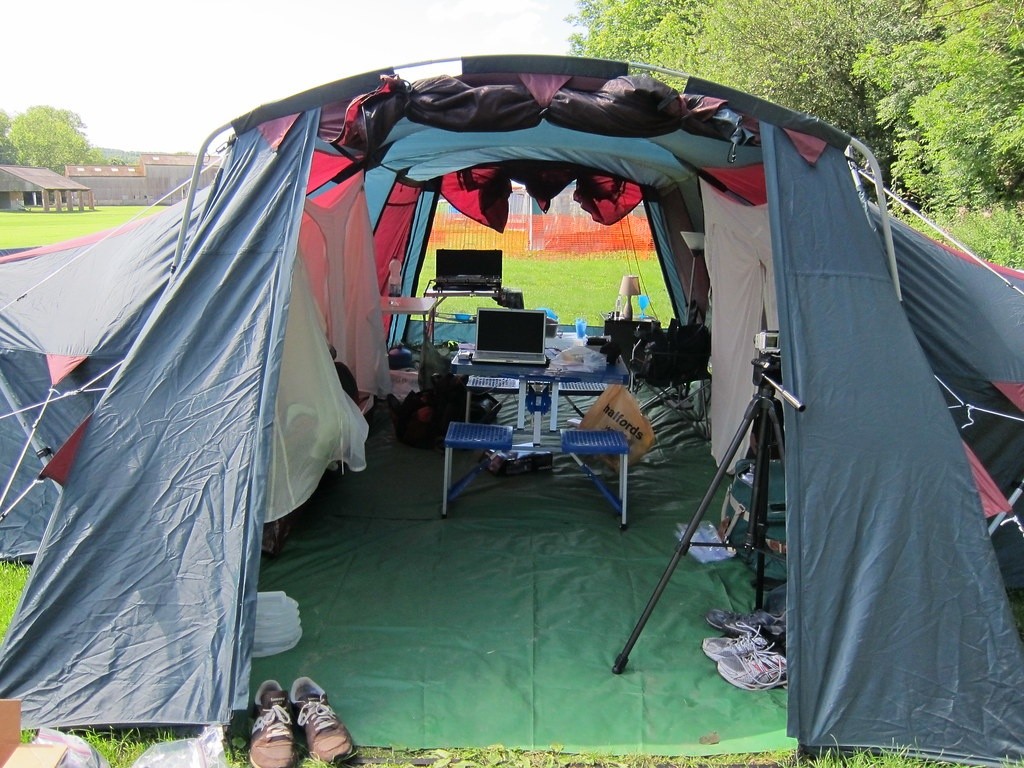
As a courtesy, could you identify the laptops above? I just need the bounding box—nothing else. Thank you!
[471,307,547,365]
[433,249,502,292]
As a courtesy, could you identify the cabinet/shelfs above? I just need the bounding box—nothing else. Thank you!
[605,317,660,372]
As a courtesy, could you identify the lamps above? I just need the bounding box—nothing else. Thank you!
[680,231,706,326]
[619,275,641,319]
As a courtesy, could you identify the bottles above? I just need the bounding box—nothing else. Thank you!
[615,296,622,320]
[388,256,401,307]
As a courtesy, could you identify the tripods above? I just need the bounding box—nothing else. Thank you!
[610,353,805,674]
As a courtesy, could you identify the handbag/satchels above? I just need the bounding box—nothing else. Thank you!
[579,384,656,471]
[718,459,787,581]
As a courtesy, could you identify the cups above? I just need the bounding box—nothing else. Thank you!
[576,316,587,339]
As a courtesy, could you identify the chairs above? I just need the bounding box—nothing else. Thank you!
[633,325,712,439]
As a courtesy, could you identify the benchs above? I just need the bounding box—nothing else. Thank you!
[465,375,608,431]
[441,421,629,531]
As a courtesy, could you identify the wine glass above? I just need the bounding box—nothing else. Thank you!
[637,296,648,318]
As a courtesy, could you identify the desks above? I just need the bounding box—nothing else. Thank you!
[423,286,500,345]
[450,349,630,445]
[379,297,438,351]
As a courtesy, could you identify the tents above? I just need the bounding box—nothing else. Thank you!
[0,47,1024,766]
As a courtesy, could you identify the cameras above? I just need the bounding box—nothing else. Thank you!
[755,333,780,354]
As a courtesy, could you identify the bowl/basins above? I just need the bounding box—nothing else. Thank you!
[455,314,470,321]
[389,344,412,368]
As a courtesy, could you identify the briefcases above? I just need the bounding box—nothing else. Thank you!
[480,442,553,476]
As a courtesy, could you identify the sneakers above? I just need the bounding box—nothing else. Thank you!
[705,608,786,640]
[701,621,787,690]
[249,677,353,767]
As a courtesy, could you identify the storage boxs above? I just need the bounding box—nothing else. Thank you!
[1,699,68,768]
